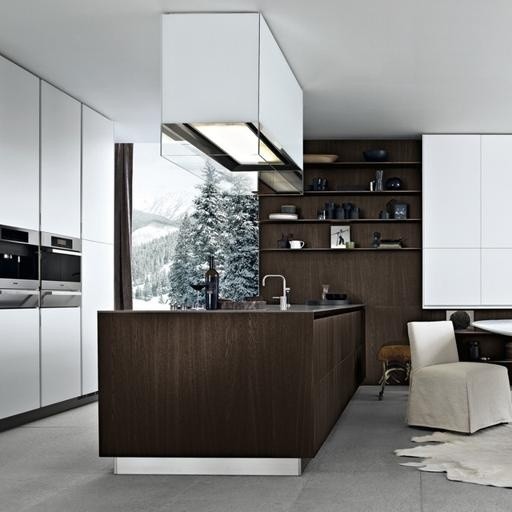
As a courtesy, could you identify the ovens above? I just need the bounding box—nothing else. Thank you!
[40,224,83,309]
[0,224,40,309]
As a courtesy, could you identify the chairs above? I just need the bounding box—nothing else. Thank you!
[404,319,512,434]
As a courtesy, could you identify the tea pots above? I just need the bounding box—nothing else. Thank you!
[289,240,306,250]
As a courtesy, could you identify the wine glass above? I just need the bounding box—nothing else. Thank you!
[187,265,205,310]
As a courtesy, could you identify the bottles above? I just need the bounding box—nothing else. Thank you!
[321,284,329,300]
[204,254,220,311]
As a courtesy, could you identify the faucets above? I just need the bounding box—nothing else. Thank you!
[261,274,287,305]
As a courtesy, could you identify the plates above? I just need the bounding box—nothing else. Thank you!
[304,299,352,305]
[268,213,298,220]
[303,154,339,163]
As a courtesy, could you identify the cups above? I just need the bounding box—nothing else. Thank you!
[315,198,361,220]
[345,242,355,249]
[277,240,289,248]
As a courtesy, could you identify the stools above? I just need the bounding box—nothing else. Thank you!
[377,341,411,402]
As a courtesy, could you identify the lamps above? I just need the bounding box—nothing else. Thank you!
[157,8,307,200]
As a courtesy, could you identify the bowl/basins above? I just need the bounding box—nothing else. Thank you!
[326,293,347,300]
[362,148,390,163]
[304,184,313,191]
[279,204,296,214]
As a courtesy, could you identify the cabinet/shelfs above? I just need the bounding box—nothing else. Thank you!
[1,49,119,436]
[253,135,421,253]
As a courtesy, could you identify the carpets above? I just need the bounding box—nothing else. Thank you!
[392,423,510,488]
